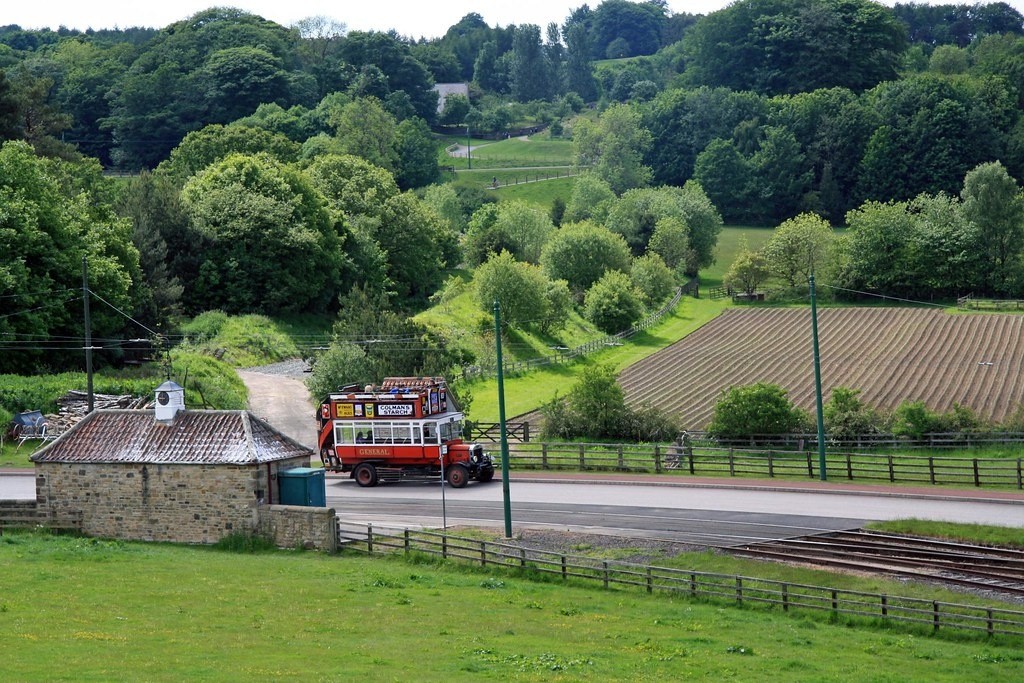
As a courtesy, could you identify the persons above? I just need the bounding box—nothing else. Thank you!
[430,380,435,384]
[391,384,410,393]
[424,425,429,437]
[356,431,373,444]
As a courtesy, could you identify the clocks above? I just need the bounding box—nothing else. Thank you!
[158,392,170,406]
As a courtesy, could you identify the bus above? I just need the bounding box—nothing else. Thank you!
[316,377,494,488]
